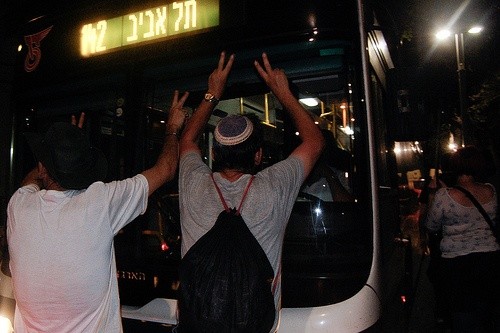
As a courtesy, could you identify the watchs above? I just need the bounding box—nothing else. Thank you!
[204,92,219,105]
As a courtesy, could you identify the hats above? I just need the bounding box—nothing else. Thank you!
[38,121,91,190]
[212,114,254,146]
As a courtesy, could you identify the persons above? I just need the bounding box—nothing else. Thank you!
[7,90,189,333]
[326,166,350,201]
[282,111,356,201]
[419,151,500,333]
[179,53,326,333]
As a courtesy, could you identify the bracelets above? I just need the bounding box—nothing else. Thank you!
[166,132,178,136]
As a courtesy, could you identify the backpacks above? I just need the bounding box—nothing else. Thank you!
[178,172,276,332]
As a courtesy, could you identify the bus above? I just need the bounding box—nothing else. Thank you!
[0,0,402,333]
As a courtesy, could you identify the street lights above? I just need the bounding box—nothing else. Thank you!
[430,22,485,146]
